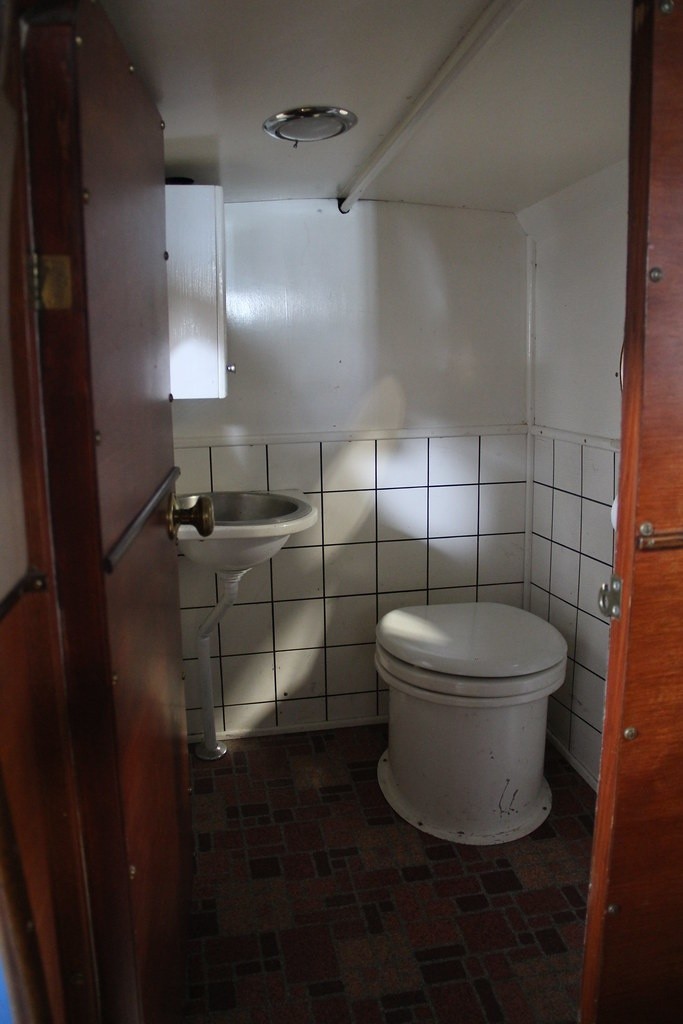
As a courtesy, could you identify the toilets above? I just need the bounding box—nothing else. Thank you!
[369,599,569,846]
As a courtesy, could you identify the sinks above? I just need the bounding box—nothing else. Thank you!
[171,487,319,572]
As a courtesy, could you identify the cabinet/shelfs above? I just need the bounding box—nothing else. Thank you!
[166,184,235,401]
